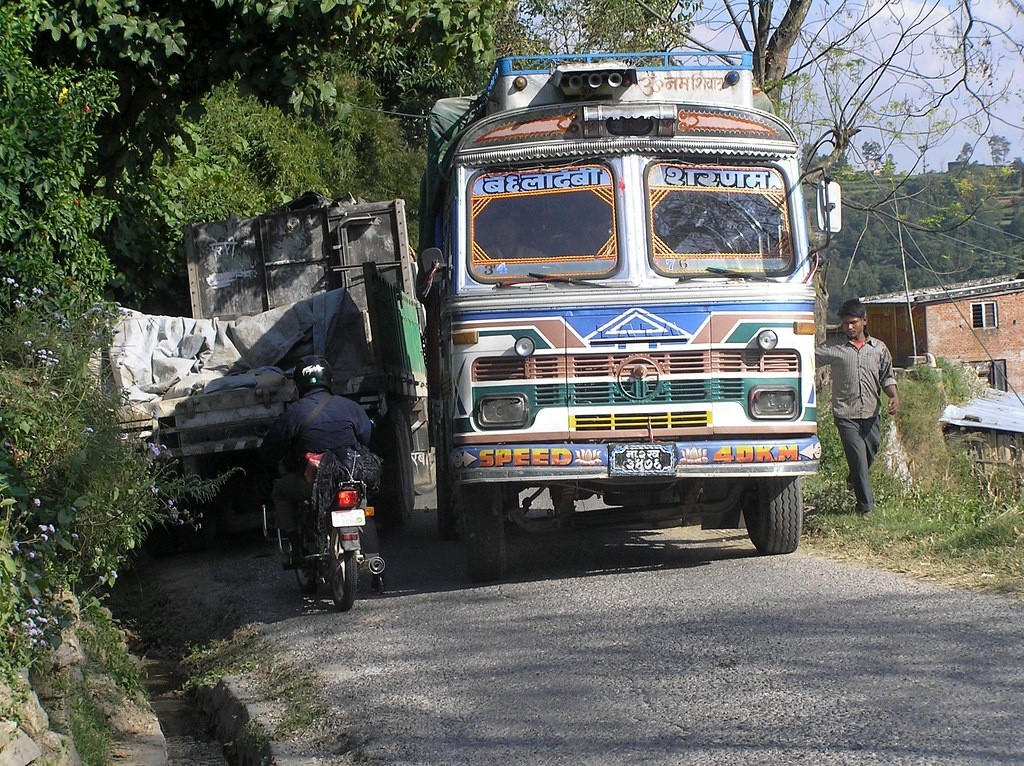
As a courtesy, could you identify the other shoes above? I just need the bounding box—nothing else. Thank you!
[370,573,387,593]
[290,545,305,563]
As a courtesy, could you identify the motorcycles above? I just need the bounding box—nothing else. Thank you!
[255,419,390,613]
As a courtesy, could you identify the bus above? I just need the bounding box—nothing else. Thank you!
[423,45,847,585]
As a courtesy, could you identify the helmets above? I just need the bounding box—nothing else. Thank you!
[293,354,334,398]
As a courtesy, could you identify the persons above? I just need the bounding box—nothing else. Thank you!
[663,204,726,255]
[483,212,550,258]
[261,355,385,594]
[816,299,899,517]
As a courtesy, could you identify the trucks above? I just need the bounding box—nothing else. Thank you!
[86,196,437,551]
